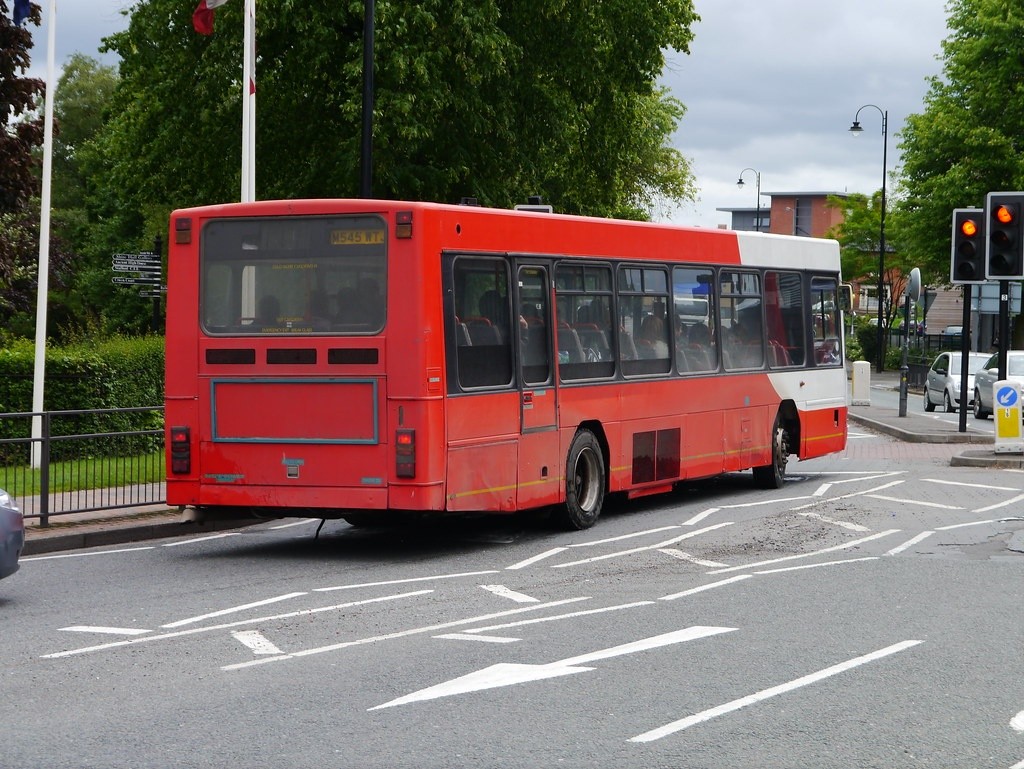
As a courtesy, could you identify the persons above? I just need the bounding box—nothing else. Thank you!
[479,289,764,346]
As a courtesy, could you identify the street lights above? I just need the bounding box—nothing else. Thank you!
[850,104,888,375]
[737,168,760,231]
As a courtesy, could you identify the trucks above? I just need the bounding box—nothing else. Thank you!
[674,297,710,329]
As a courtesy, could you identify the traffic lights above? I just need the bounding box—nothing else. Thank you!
[985,191,1024,281]
[951,207,986,285]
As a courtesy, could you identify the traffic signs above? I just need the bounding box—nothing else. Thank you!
[111,252,163,303]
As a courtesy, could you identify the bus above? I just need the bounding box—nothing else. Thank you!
[163,197,857,531]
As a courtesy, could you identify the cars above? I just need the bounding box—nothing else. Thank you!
[924,353,994,413]
[868,317,889,329]
[898,314,923,333]
[576,288,594,311]
[941,324,972,351]
[812,300,835,312]
[0,491,26,579]
[974,351,1024,419]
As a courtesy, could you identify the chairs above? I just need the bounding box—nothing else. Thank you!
[453,306,795,373]
